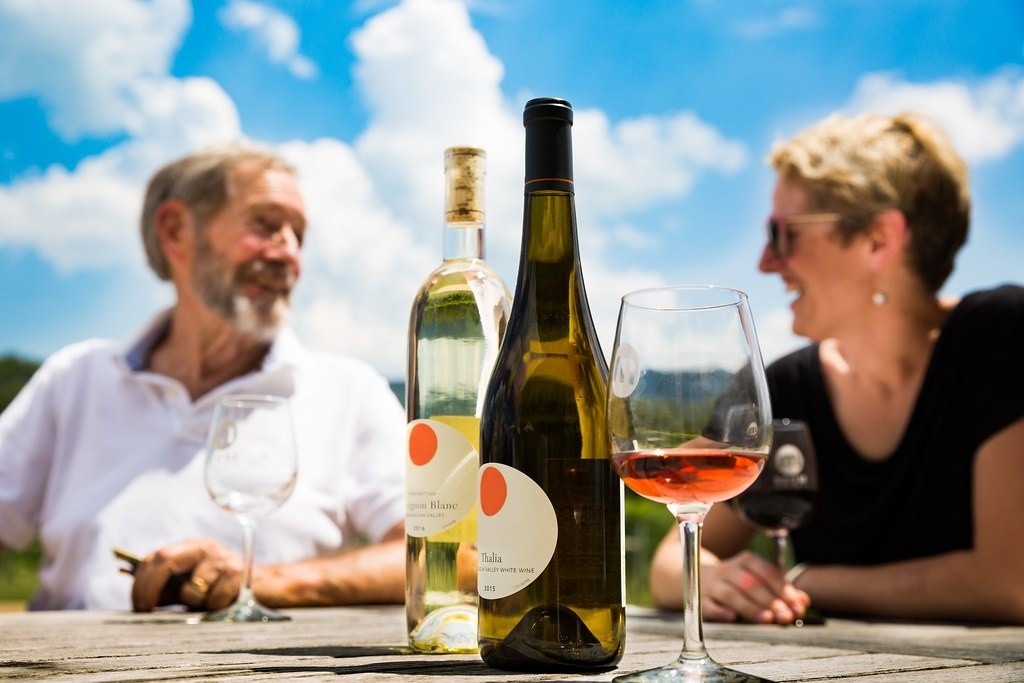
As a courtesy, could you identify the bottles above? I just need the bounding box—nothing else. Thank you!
[479,99,626,673]
[403,147,518,655]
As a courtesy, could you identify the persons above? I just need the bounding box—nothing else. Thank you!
[651,109,1024,625]
[0,147,481,611]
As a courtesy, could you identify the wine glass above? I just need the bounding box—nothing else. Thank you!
[184,393,299,624]
[724,418,826,627]
[606,285,778,683]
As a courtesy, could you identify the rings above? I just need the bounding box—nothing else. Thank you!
[189,574,210,593]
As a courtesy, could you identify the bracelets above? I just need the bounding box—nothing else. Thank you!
[783,563,808,584]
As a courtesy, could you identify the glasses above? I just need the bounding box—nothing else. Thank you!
[769,214,842,264]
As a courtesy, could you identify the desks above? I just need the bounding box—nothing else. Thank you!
[0,604,1024,683]
